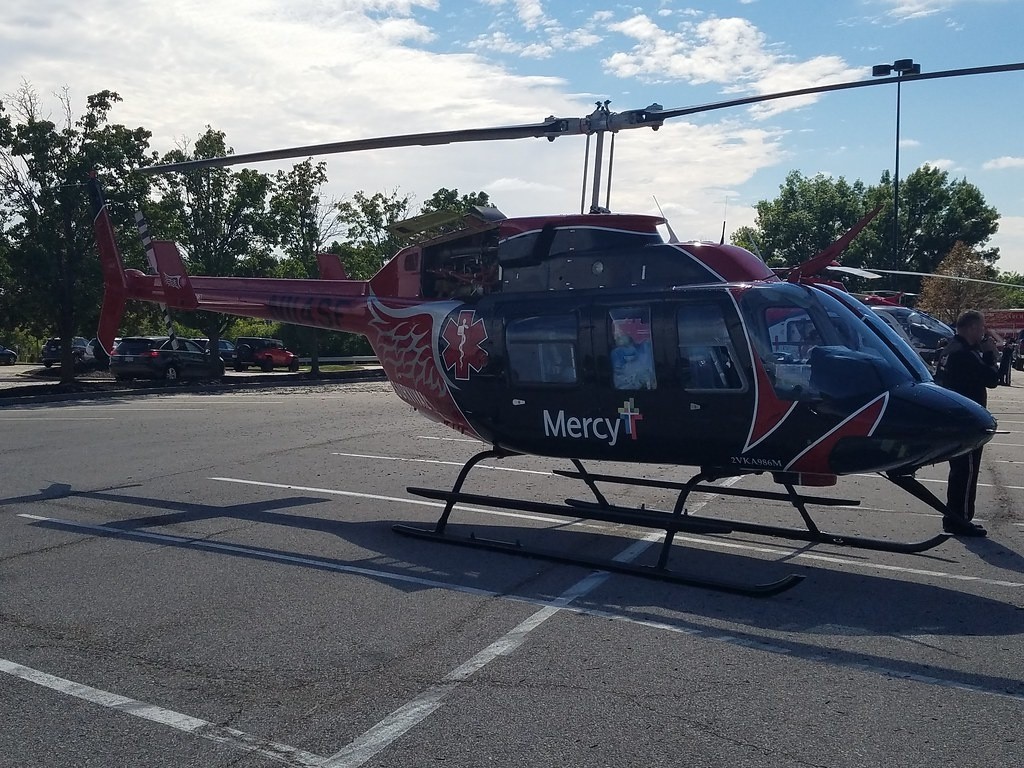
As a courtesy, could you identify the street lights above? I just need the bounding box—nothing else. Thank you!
[871,58,922,292]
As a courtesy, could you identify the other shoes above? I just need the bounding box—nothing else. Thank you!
[942,518,988,537]
[998,381,1005,385]
[1001,383,1010,386]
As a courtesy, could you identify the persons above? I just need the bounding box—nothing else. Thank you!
[933,309,1001,538]
[1001,337,1024,387]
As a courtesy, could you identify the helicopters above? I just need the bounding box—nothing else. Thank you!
[618,204,958,377]
[89,56,1024,603]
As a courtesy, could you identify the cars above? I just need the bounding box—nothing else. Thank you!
[0,345,18,365]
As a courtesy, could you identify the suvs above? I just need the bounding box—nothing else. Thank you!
[235,336,303,372]
[82,337,123,368]
[41,336,90,368]
[175,338,236,367]
[108,334,227,383]
